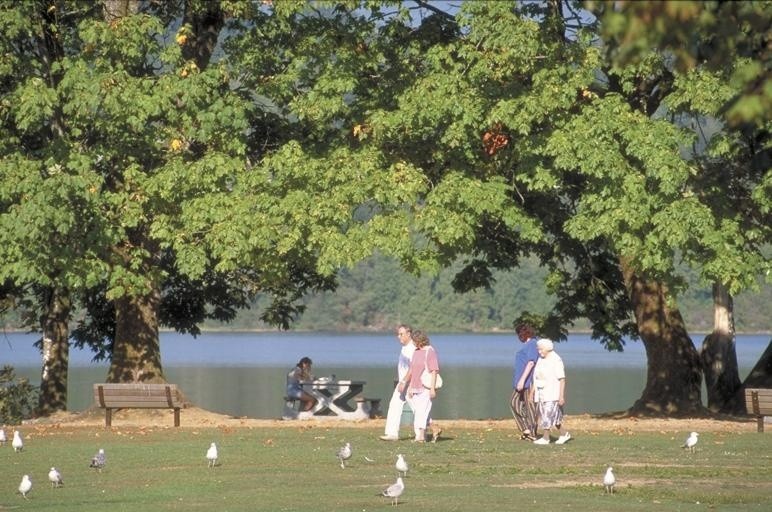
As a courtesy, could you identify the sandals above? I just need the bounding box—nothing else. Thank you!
[410,440,427,444]
[431,426,442,443]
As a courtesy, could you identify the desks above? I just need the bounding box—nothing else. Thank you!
[299,380,367,421]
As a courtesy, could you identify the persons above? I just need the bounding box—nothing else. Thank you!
[509,323,539,441]
[286,357,316,411]
[379,323,418,442]
[528,338,572,445]
[407,330,443,443]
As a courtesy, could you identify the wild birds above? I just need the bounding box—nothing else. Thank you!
[14,474,33,500]
[206,442,218,469]
[383,477,405,507]
[89,448,106,475]
[395,454,410,478]
[603,466,616,495]
[11,431,23,453]
[48,467,64,488]
[335,442,353,470]
[0,424,7,448]
[680,431,700,454]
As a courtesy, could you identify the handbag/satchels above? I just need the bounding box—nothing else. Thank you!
[420,368,443,389]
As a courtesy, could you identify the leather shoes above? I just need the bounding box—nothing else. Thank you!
[379,435,399,442]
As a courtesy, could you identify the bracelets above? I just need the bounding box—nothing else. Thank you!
[400,381,406,386]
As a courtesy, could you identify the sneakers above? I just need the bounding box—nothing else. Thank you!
[520,433,551,445]
[554,432,572,444]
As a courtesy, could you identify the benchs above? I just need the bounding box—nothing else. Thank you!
[744,387,772,433]
[94,383,181,427]
[281,397,301,420]
[356,398,380,420]
